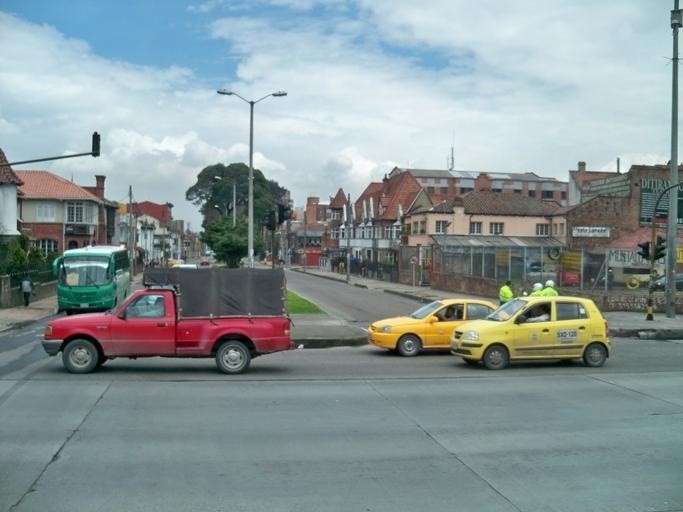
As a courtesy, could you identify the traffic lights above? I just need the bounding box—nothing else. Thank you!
[654,236,667,261]
[637,241,649,259]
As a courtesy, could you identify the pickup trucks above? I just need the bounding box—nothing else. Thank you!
[41,266,293,374]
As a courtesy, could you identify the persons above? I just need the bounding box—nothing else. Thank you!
[525,304,550,322]
[436,305,458,321]
[148,258,159,267]
[499,280,513,307]
[18,271,34,306]
[527,283,544,297]
[606,268,614,289]
[135,297,164,318]
[538,280,559,296]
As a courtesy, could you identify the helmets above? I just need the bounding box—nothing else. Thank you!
[545,279,554,287]
[532,283,543,291]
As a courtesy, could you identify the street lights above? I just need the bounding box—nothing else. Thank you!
[213,175,255,226]
[216,87,288,267]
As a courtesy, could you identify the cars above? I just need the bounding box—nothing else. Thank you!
[653,273,683,291]
[368,299,510,358]
[451,294,610,370]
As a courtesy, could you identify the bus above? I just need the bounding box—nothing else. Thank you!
[51,244,130,315]
